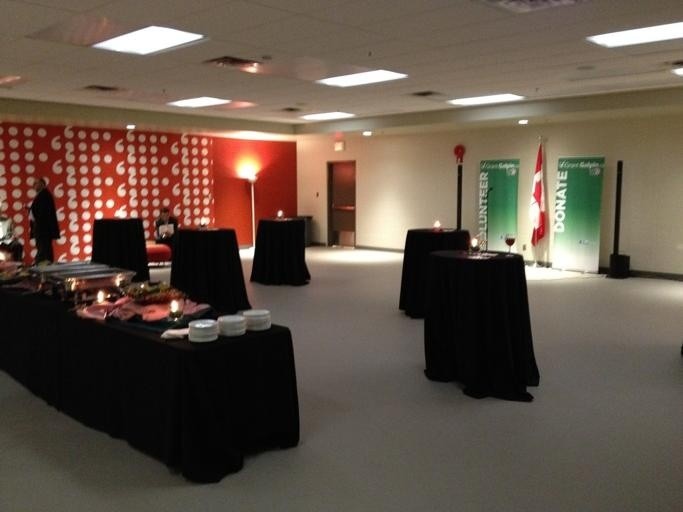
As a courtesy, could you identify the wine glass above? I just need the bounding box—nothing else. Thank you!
[504,233,516,257]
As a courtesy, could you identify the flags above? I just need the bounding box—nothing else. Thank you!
[528,141,546,246]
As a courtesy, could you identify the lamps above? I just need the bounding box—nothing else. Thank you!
[248,176,258,248]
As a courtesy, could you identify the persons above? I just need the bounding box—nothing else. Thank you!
[156,208,178,244]
[29,178,60,266]
[1,217,23,262]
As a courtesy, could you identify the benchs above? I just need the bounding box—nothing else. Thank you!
[146,244,171,265]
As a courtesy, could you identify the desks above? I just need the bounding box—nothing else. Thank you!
[250,217,311,285]
[424,251,540,403]
[92,218,150,281]
[169,226,252,314]
[399,228,470,319]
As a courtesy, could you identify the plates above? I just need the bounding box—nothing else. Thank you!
[187,309,271,343]
